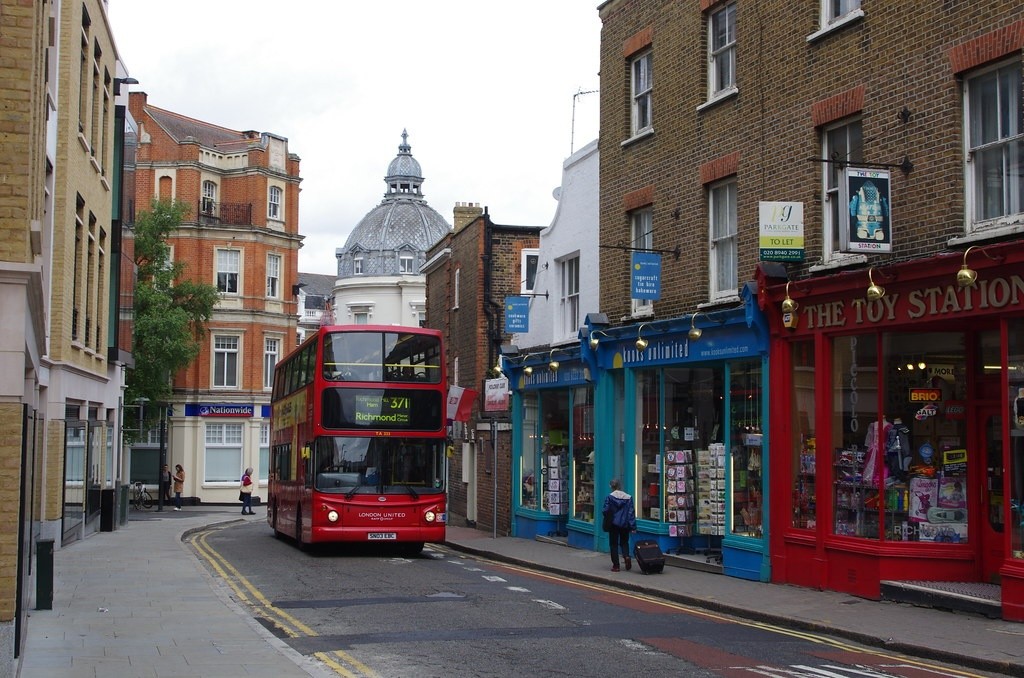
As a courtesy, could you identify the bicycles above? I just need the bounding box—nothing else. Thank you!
[133,482,153,511]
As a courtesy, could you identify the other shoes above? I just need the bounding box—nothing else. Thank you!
[625,556,632,571]
[249,512,256,515]
[611,564,620,572]
[174,508,182,510]
[242,511,248,515]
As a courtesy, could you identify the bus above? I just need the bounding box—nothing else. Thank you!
[267,325,454,556]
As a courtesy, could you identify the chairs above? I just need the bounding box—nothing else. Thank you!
[323,362,431,382]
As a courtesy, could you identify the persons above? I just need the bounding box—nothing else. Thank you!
[240,467,256,515]
[364,458,392,485]
[172,464,185,510]
[950,482,964,500]
[602,480,637,571]
[163,464,173,505]
[861,415,912,481]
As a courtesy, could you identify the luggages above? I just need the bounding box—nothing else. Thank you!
[631,531,665,575]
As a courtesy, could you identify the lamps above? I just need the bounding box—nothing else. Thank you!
[955,246,1005,288]
[492,355,521,376]
[549,348,574,373]
[590,329,621,351]
[865,266,897,300]
[687,310,726,341]
[529,432,544,439]
[896,352,927,371]
[780,278,811,313]
[635,323,669,351]
[523,352,545,378]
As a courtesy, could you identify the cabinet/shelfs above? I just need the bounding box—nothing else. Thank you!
[797,472,817,529]
[580,461,593,507]
[834,449,863,531]
[856,480,909,540]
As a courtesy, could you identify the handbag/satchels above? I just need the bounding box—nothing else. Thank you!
[602,509,614,532]
[239,493,243,500]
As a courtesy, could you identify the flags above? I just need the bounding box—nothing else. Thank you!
[447,384,480,422]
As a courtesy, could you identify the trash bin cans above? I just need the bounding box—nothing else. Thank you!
[36,538,54,610]
[100,488,117,532]
[89,484,101,514]
[120,484,131,527]
